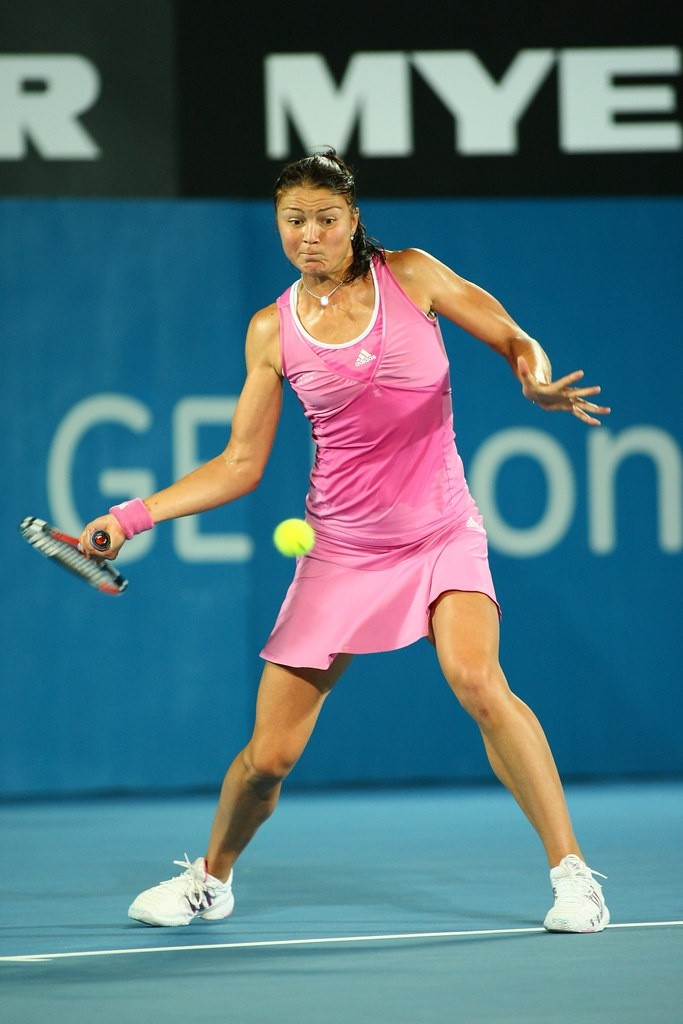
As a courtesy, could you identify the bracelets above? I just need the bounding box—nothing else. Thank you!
[108,497,154,538]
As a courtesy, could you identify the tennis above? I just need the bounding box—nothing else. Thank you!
[273,518,315,558]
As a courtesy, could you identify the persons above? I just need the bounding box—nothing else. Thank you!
[80,144,617,933]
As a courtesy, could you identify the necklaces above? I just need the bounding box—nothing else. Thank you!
[302,273,342,305]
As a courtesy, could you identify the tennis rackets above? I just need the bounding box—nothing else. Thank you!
[19,515,129,596]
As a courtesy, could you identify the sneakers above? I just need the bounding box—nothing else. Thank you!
[128,852,234,926]
[543,854,610,933]
